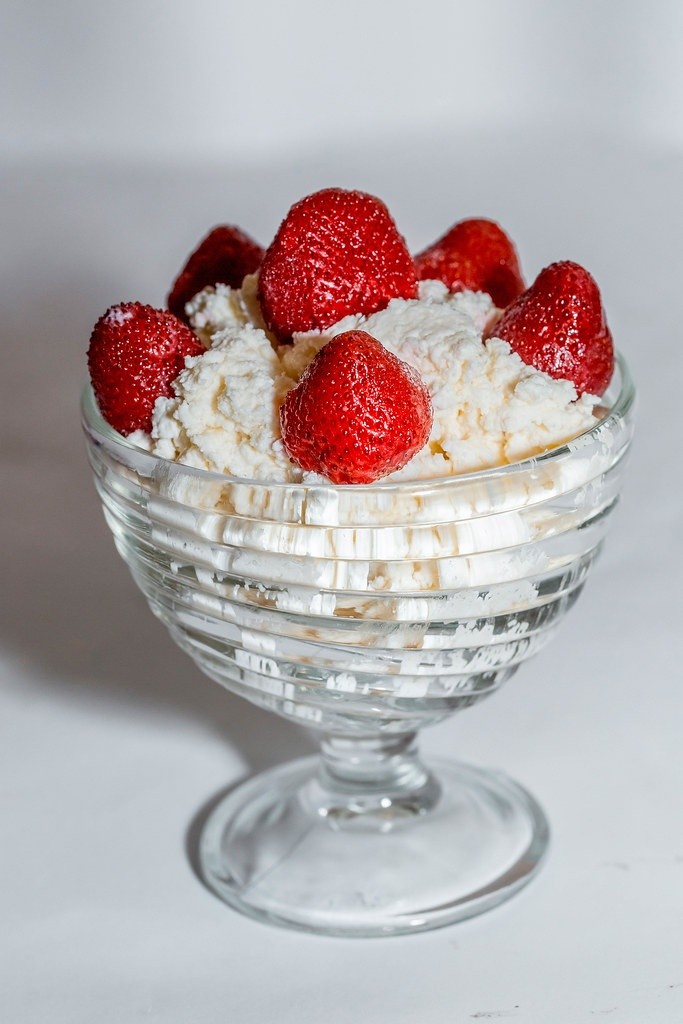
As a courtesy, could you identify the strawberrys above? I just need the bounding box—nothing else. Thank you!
[88,188,616,483]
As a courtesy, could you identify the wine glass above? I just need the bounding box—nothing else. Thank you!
[75,348,640,939]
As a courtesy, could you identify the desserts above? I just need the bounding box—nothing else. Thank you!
[127,264,611,720]
